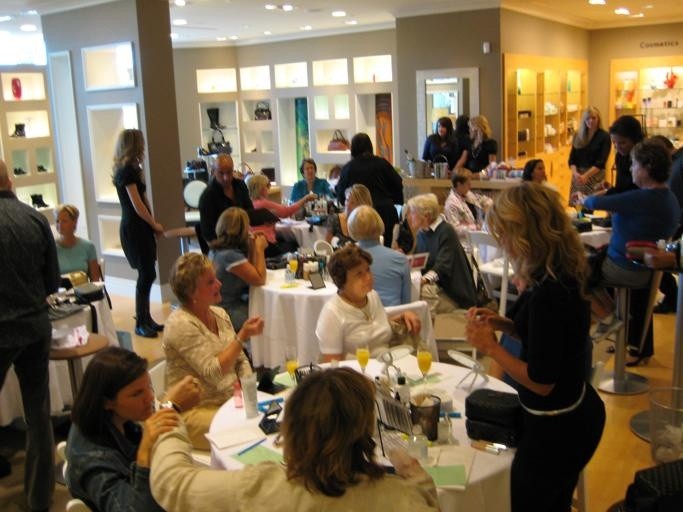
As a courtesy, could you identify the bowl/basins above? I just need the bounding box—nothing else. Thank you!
[51,329,70,347]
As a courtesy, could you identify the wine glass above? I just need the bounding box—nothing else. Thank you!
[417,343,434,390]
[284,345,298,384]
[354,341,370,377]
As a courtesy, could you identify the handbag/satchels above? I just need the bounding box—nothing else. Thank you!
[74,282,105,306]
[186,159,208,183]
[326,128,350,151]
[207,128,231,155]
[232,161,255,181]
[463,387,523,448]
[252,101,271,121]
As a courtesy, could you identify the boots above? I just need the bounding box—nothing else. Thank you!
[29,194,48,209]
[205,107,225,130]
[9,123,25,138]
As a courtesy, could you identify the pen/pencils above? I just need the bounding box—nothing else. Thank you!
[237,438,267,455]
[259,398,283,406]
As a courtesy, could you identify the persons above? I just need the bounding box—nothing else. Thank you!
[607,457,682,512]
[0,161,61,512]
[522,160,546,186]
[569,106,682,366]
[53,204,99,284]
[149,103,496,511]
[111,129,164,338]
[65,346,179,511]
[464,182,607,512]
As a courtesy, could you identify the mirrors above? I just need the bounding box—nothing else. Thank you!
[415,67,480,163]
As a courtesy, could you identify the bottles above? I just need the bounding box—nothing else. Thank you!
[393,376,411,404]
[232,383,243,409]
[284,247,329,284]
[436,411,453,444]
[408,423,430,466]
[281,196,327,221]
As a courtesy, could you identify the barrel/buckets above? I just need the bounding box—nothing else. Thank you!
[407,158,425,179]
[432,154,449,180]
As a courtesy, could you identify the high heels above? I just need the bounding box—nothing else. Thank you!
[605,344,653,366]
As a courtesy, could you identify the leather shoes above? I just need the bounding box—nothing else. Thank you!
[13,165,48,175]
[133,316,164,338]
[651,289,676,315]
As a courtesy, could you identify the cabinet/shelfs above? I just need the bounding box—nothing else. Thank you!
[607,55,683,190]
[0,66,63,229]
[193,54,398,203]
[502,52,587,207]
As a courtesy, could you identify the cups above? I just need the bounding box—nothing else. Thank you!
[292,364,323,385]
[239,372,259,419]
[409,393,442,441]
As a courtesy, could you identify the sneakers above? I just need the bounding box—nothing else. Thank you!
[589,315,623,342]
